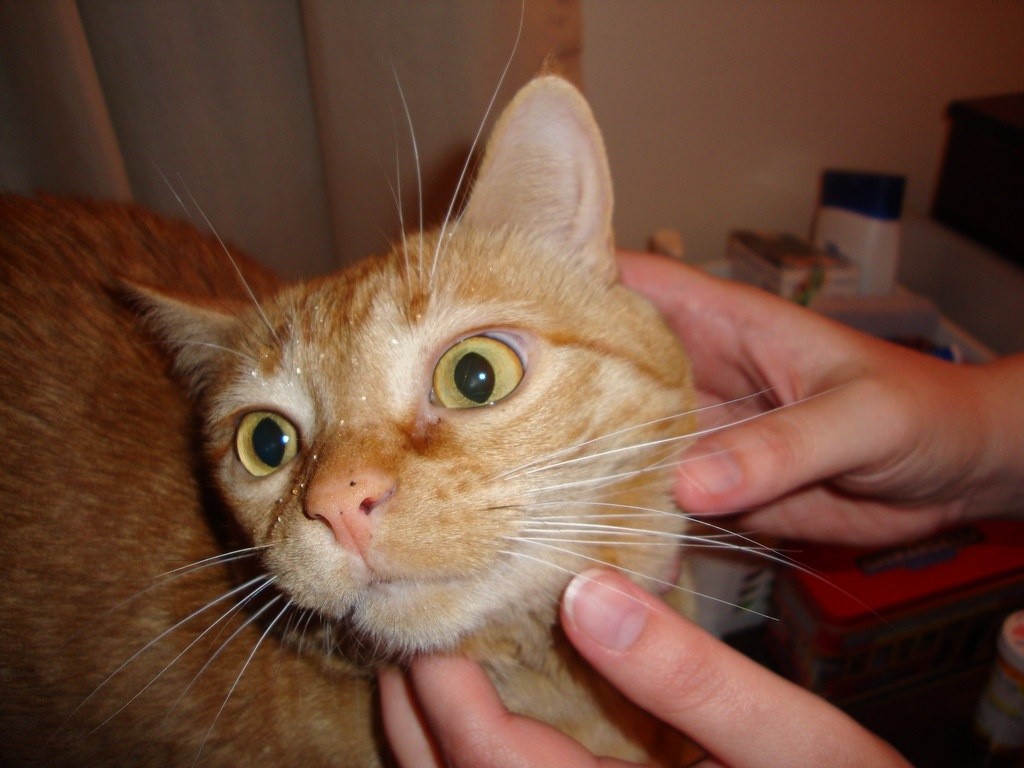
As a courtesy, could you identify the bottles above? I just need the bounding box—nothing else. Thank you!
[973,611,1024,750]
[815,165,908,295]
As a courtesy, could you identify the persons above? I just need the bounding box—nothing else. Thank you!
[378,249,1024,768]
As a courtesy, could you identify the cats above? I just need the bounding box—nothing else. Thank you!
[1,75,701,768]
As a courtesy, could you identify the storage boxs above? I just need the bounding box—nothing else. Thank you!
[769,519,1024,708]
